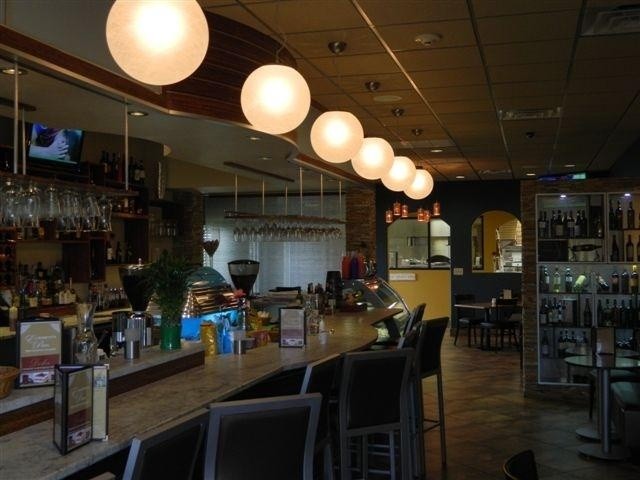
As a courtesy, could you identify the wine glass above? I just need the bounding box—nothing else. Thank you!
[231,218,343,243]
[0,180,112,234]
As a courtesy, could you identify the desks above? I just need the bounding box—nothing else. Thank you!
[563,355,640,466]
[0,307,406,480]
[0,304,152,369]
[563,346,640,443]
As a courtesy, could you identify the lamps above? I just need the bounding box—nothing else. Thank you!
[237,62,312,137]
[402,127,434,202]
[308,40,364,164]
[379,107,416,193]
[384,200,442,224]
[102,0,212,86]
[350,80,395,181]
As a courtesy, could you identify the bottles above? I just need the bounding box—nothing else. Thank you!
[538,200,640,384]
[342,250,366,279]
[116,241,122,262]
[0,260,77,331]
[105,234,113,263]
[88,282,130,312]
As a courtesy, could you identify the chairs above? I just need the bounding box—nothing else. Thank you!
[451,293,524,354]
[501,448,541,480]
[296,352,341,480]
[370,302,428,351]
[91,406,208,480]
[329,346,417,480]
[410,315,451,437]
[203,392,323,479]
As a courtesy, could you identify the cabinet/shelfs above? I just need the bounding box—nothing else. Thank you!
[534,191,640,389]
[340,275,411,333]
[0,171,175,328]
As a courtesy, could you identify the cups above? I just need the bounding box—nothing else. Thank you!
[112,311,128,352]
[124,318,140,359]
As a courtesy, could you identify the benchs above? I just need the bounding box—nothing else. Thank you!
[610,381,638,449]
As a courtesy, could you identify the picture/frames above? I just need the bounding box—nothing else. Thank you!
[277,305,308,349]
[14,316,65,391]
